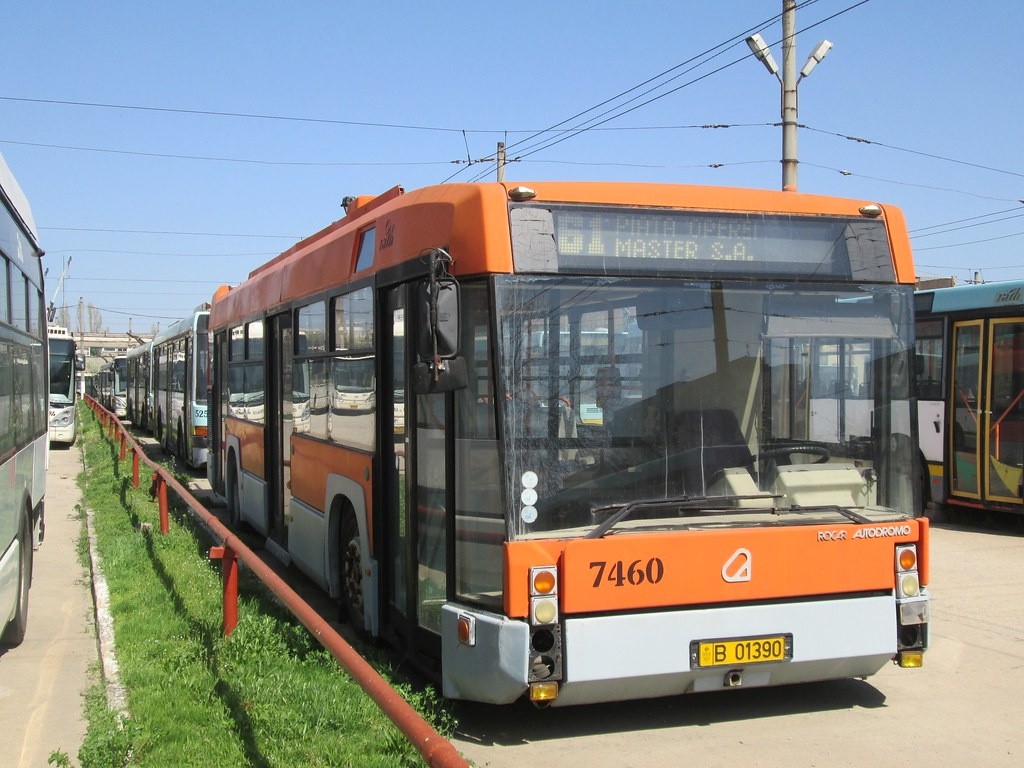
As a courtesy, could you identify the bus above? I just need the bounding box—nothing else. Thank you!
[0,147,53,659]
[90,312,311,473]
[771,276,1024,525]
[44,324,85,445]
[331,347,412,439]
[206,177,935,715]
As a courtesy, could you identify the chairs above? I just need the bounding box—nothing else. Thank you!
[793,377,976,405]
[671,408,759,497]
